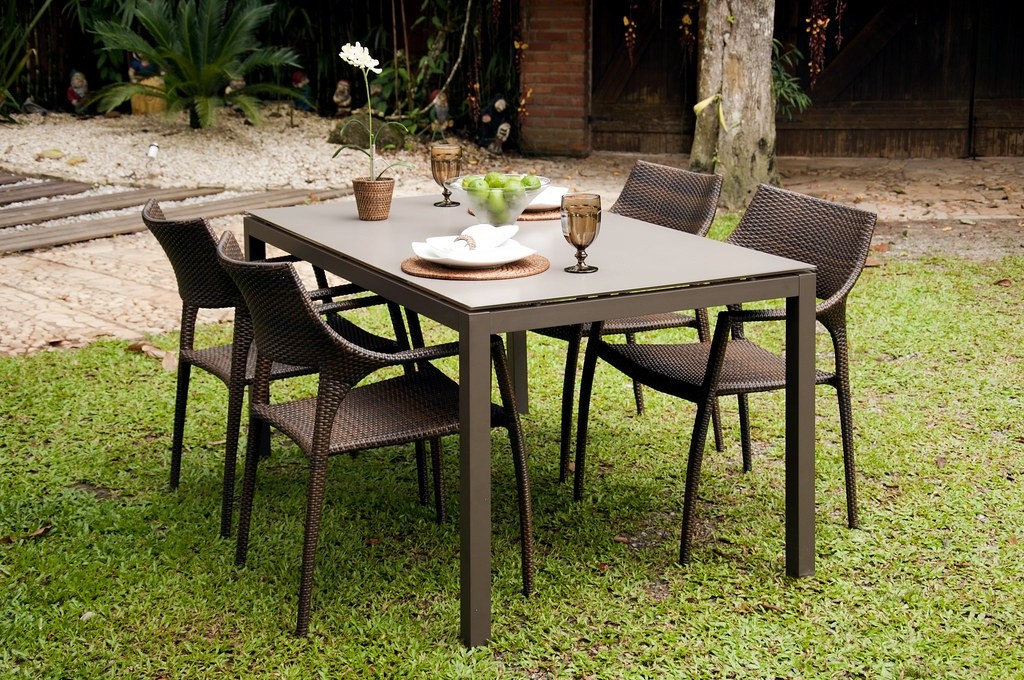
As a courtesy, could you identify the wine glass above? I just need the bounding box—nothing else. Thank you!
[431,146,460,207]
[561,194,601,272]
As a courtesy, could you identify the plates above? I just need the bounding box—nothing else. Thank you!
[524,187,570,210]
[412,241,536,266]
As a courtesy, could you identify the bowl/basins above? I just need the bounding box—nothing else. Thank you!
[446,173,551,227]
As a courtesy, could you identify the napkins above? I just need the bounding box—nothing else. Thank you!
[531,185,569,203]
[449,224,520,253]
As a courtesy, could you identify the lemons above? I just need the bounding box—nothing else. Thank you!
[462,172,541,224]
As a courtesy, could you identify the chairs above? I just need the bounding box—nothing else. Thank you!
[141,197,430,539]
[215,229,536,638]
[574,183,878,564]
[521,157,728,484]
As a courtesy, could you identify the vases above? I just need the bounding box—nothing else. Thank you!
[351,177,395,221]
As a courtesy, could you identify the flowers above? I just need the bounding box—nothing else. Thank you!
[331,41,418,180]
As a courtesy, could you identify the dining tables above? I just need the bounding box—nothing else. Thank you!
[243,180,818,651]
